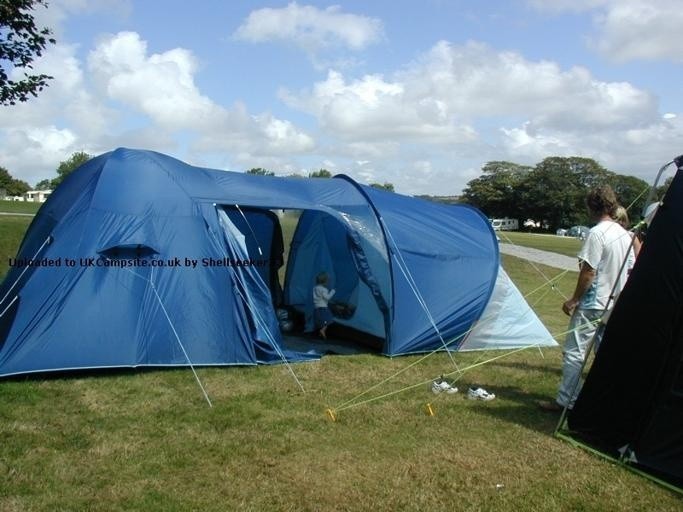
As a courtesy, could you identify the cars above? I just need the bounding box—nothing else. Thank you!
[564,225,590,237]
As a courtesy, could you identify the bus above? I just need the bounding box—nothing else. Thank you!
[491,219,519,231]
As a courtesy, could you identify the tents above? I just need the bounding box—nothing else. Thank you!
[555,156,683,494]
[1,147,500,377]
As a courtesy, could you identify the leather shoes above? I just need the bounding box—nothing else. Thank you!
[532,396,565,414]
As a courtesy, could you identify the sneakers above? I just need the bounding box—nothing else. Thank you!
[430,380,458,395]
[466,387,496,402]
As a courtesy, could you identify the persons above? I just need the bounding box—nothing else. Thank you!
[613,205,641,260]
[312,271,336,339]
[533,183,636,411]
